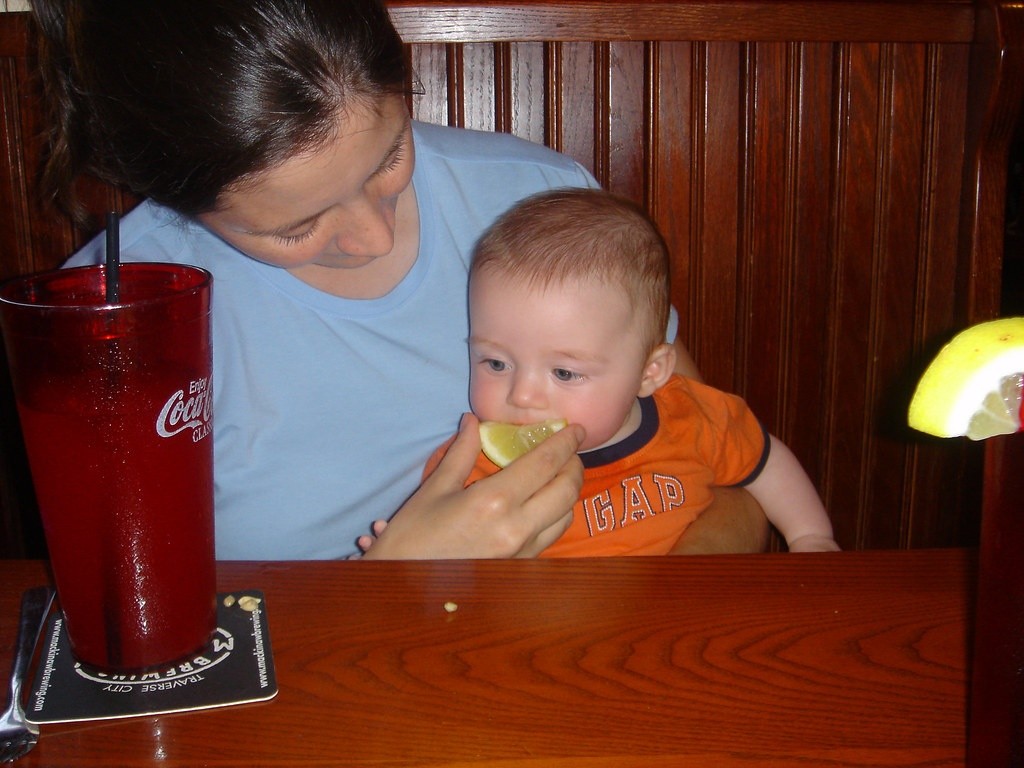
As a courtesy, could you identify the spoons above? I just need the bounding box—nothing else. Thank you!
[0,585,56,764]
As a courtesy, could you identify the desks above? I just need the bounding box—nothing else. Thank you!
[5,550,1024,768]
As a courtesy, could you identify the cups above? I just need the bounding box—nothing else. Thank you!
[0,263,213,670]
[965,432,1024,767]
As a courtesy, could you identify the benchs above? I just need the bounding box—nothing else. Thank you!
[0,0,1023,554]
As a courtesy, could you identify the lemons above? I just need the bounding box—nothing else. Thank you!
[477,420,568,470]
[907,317,1024,440]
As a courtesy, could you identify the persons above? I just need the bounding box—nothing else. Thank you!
[344,186,845,561]
[59,1,772,562]
